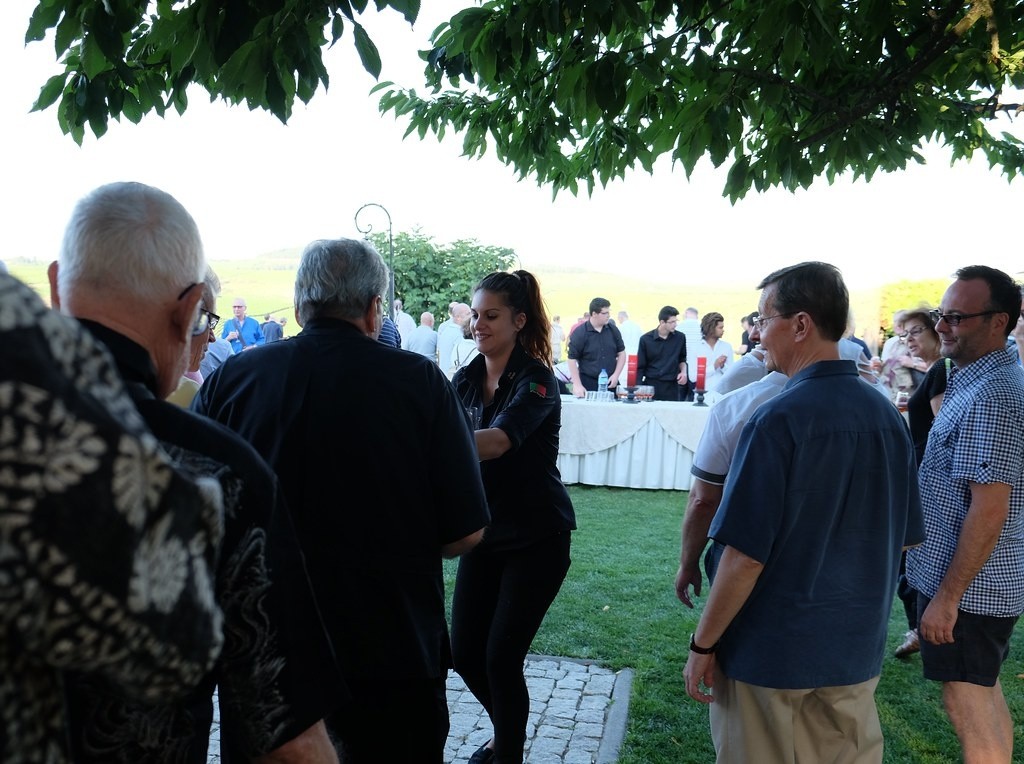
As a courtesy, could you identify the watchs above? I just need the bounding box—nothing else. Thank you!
[689,633,715,655]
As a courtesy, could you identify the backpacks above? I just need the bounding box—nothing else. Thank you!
[447,342,477,383]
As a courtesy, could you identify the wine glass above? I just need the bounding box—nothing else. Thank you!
[616,386,655,402]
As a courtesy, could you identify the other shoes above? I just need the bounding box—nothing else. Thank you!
[468,736,497,764]
[894,630,921,659]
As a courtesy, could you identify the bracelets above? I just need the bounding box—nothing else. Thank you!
[913,359,917,370]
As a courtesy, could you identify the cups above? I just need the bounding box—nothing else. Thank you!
[584,390,615,402]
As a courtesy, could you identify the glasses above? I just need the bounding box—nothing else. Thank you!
[929,309,1005,326]
[232,304,243,309]
[752,311,799,335]
[665,320,678,324]
[199,307,220,331]
[898,325,930,341]
[599,311,610,316]
[177,283,209,337]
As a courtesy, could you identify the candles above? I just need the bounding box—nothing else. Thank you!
[627,355,637,387]
[696,357,706,389]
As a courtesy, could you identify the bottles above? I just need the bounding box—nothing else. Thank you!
[598,368,608,392]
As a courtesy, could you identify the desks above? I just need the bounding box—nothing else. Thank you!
[556,395,714,491]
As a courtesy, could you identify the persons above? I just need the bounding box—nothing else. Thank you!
[682,261,928,764]
[673,371,790,609]
[377,300,482,383]
[189,238,492,764]
[905,266,1024,764]
[0,182,337,764]
[895,308,954,658]
[450,270,577,764]
[544,298,1024,402]
